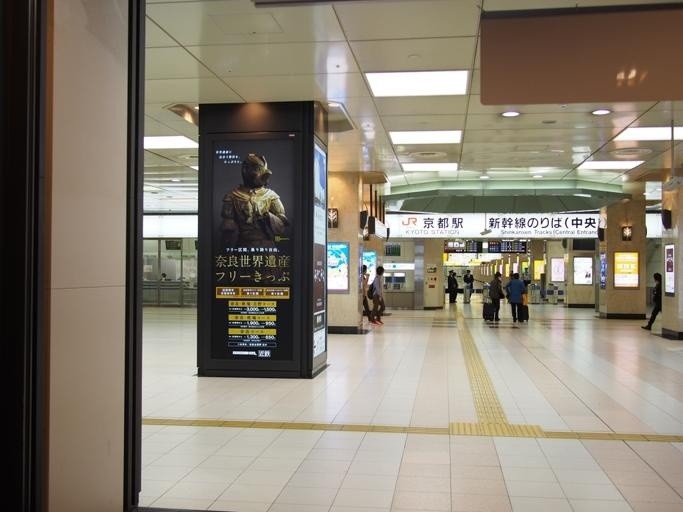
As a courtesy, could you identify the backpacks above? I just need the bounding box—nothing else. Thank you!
[367,284,373,299]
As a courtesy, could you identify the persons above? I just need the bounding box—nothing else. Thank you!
[363,265,385,325]
[489,268,531,322]
[641,273,662,330]
[448,270,474,303]
[221,154,288,247]
[160,273,166,281]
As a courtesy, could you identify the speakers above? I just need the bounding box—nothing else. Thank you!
[368,216,375,234]
[360,210,369,229]
[662,209,672,229]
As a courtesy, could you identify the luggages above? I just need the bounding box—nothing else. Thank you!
[518,291,530,323]
[483,296,495,321]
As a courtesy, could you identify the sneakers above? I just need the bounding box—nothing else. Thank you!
[641,324,652,331]
[372,319,384,325]
